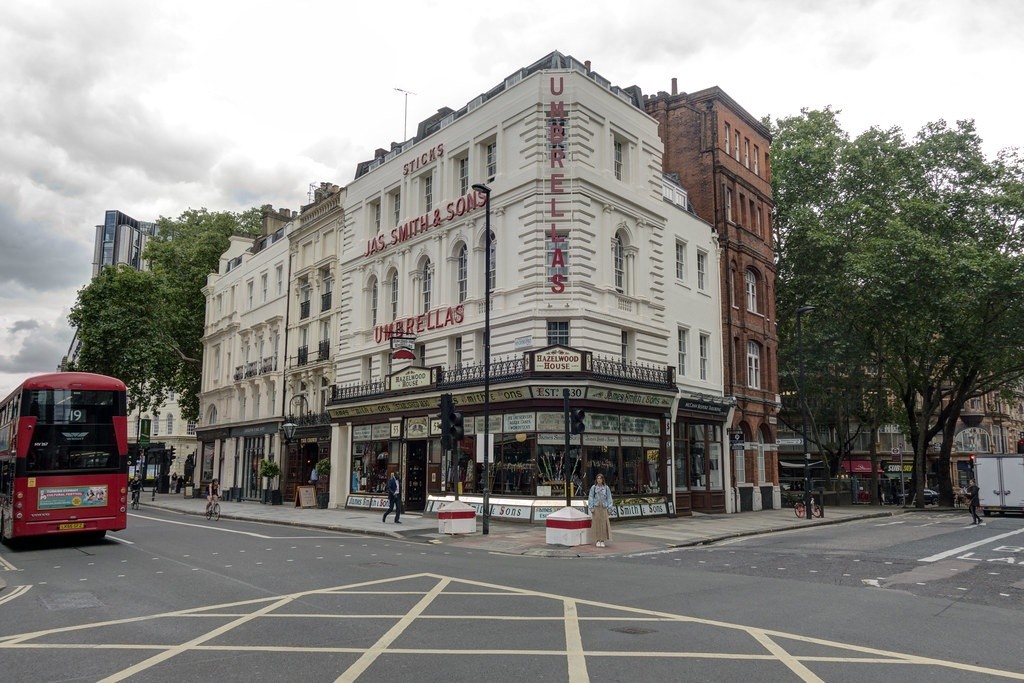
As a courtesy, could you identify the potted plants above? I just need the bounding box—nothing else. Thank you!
[315,458,331,509]
[260,459,281,504]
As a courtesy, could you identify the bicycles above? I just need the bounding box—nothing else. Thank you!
[206,495,223,522]
[793,490,823,518]
[131,490,144,510]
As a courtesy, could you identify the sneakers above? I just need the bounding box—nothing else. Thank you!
[596,541,601,548]
[601,542,605,548]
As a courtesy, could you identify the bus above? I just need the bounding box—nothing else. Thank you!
[0,371,131,543]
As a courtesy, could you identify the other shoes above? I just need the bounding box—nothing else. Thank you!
[969,521,976,525]
[382,515,386,522]
[394,520,402,524]
[978,519,983,525]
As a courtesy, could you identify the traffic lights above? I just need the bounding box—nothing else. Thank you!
[970,455,974,468]
[144,446,151,457]
[137,442,142,460]
[170,445,177,462]
[447,410,465,441]
[437,393,454,450]
[570,410,586,434]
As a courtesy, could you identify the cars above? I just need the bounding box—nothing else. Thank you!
[898,489,940,505]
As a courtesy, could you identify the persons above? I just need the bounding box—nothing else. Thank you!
[205,478,223,513]
[802,488,825,519]
[958,485,972,508]
[382,471,404,524]
[154,473,162,491]
[878,484,901,506]
[169,473,183,495]
[129,471,145,508]
[588,473,617,548]
[963,479,984,525]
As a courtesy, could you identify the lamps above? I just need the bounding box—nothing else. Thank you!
[280,416,298,444]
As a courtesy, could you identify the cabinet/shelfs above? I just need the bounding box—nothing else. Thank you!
[542,481,574,498]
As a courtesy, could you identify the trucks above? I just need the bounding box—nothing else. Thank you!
[972,452,1024,517]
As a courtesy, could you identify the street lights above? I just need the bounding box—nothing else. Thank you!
[794,304,818,521]
[469,183,491,535]
